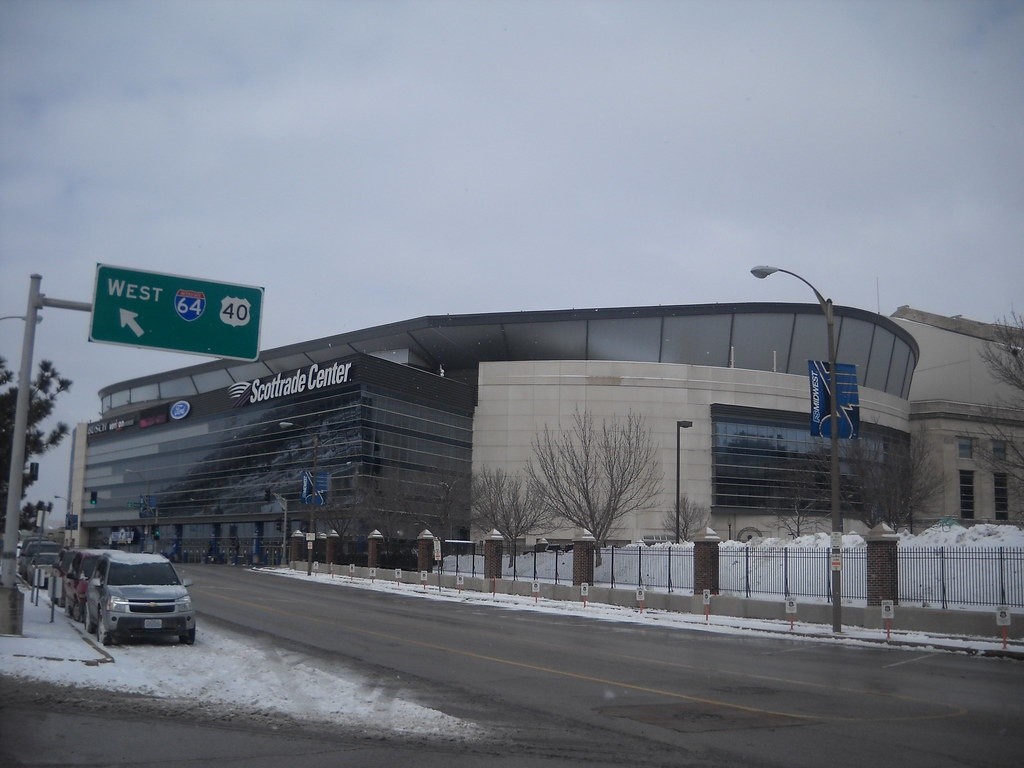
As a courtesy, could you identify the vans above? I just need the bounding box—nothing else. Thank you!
[642,535,676,547]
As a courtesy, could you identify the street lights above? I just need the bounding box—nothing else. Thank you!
[279,421,314,576]
[676,420,694,545]
[126,469,149,553]
[750,265,844,633]
[54,496,74,547]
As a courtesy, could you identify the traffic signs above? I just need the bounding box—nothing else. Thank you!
[85,262,266,365]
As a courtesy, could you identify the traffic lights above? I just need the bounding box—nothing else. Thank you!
[91,491,98,504]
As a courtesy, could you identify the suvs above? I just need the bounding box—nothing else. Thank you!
[18,535,126,621]
[86,550,198,646]
